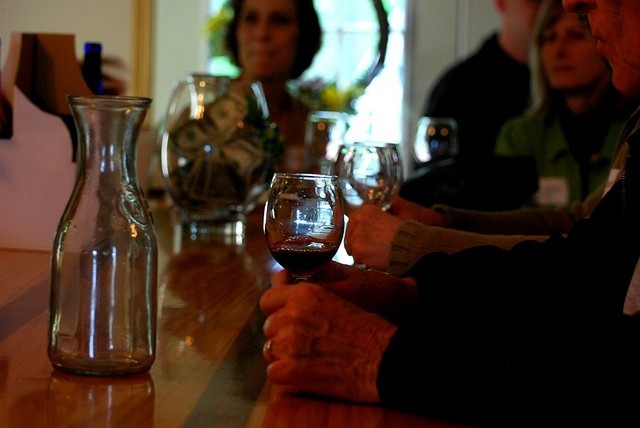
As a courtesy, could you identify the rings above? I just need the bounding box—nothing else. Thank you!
[266,339,276,360]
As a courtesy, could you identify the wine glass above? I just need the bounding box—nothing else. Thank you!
[305,112,347,176]
[330,144,397,218]
[350,142,404,195]
[413,118,456,167]
[263,172,345,283]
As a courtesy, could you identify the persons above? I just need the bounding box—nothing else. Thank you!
[497,0,628,210]
[343,137,632,277]
[202,0,328,215]
[413,0,553,172]
[261,1,639,416]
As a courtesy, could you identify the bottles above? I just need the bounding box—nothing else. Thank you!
[46,94,158,377]
